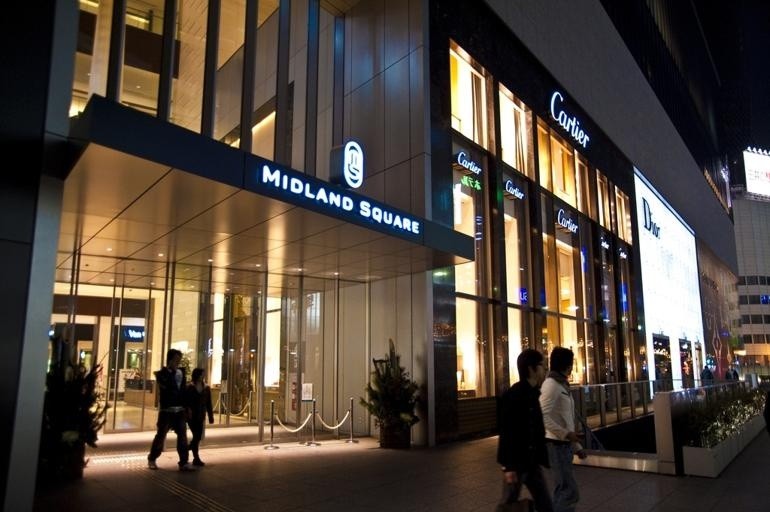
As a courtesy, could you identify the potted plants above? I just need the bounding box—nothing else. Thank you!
[358,338,426,450]
[45,345,115,484]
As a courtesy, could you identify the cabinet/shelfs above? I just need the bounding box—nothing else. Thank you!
[124,378,158,410]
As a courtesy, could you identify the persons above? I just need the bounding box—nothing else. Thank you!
[494,348,555,512]
[187,367,214,467]
[540,346,587,512]
[700,366,714,401]
[725,365,738,382]
[148,349,195,472]
